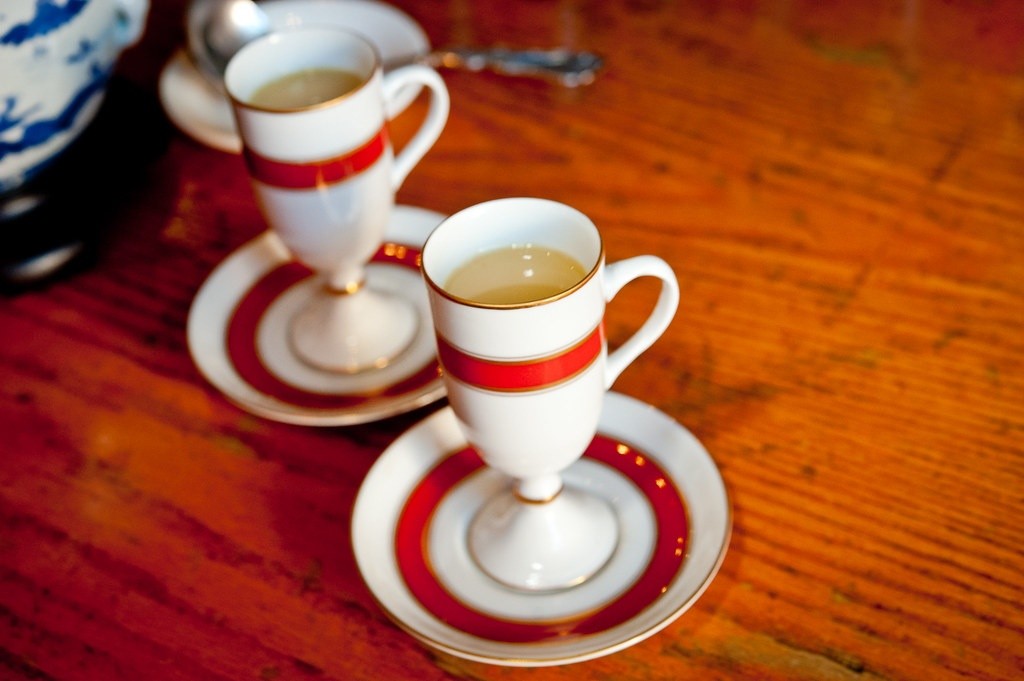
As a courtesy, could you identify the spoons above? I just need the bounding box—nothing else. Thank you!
[184,1,604,98]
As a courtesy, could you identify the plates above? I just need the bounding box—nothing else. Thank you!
[158,0,429,153]
[187,205,455,426]
[349,390,734,670]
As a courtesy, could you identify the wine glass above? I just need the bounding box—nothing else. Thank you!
[421,196,681,590]
[221,26,450,374]
